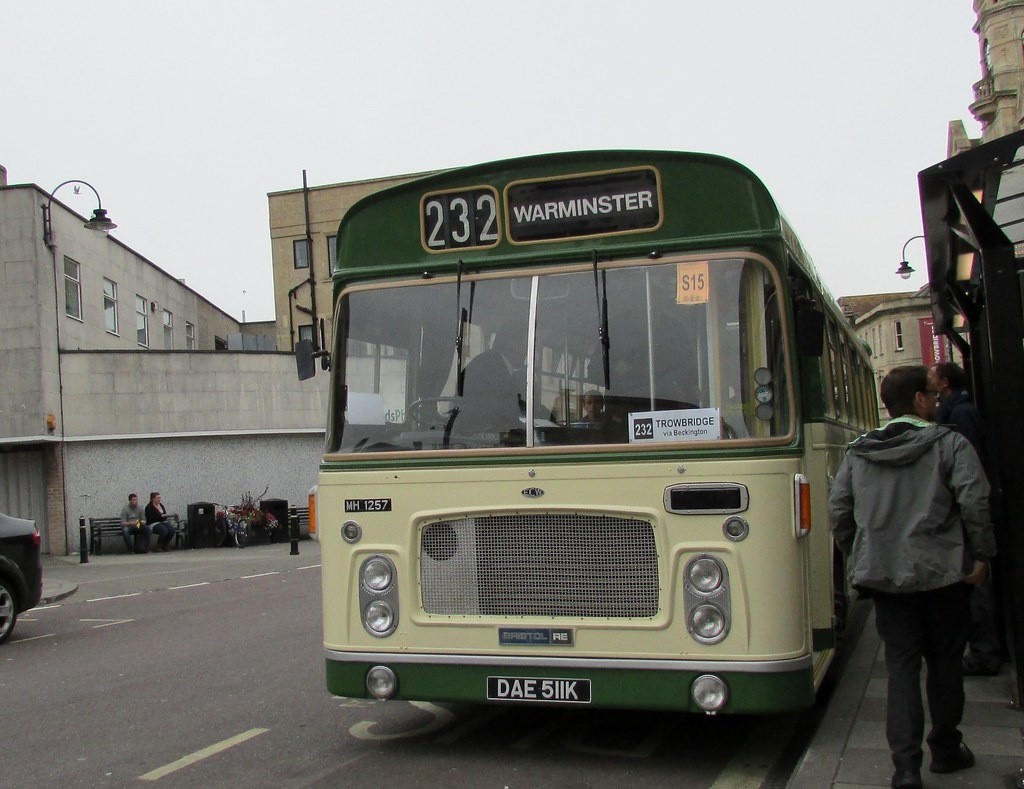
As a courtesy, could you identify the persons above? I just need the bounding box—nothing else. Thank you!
[828,360,1003,789]
[448,320,604,432]
[120,493,152,555]
[145,492,174,553]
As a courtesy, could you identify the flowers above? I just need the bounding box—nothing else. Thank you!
[214,494,280,531]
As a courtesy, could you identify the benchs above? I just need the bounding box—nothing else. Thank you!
[288,507,310,526]
[89,514,190,557]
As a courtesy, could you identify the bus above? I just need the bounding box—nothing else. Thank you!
[292,147,882,722]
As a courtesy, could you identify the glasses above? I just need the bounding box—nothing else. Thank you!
[929,390,940,398]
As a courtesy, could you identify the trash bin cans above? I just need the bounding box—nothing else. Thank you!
[260,499,288,546]
[187,502,216,548]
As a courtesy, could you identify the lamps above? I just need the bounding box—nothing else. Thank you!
[40,179,118,248]
[894,236,925,280]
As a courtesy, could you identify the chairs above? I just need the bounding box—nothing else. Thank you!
[453,353,521,431]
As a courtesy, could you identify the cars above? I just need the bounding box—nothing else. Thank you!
[0,512,42,644]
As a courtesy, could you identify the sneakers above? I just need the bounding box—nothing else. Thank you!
[892,768,922,789]
[930,748,975,773]
[963,654,1000,675]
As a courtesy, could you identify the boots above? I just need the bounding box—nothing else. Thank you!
[156,543,171,551]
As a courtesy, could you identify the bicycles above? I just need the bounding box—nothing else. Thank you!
[212,501,251,548]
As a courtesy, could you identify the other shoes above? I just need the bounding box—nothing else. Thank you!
[128,549,135,553]
